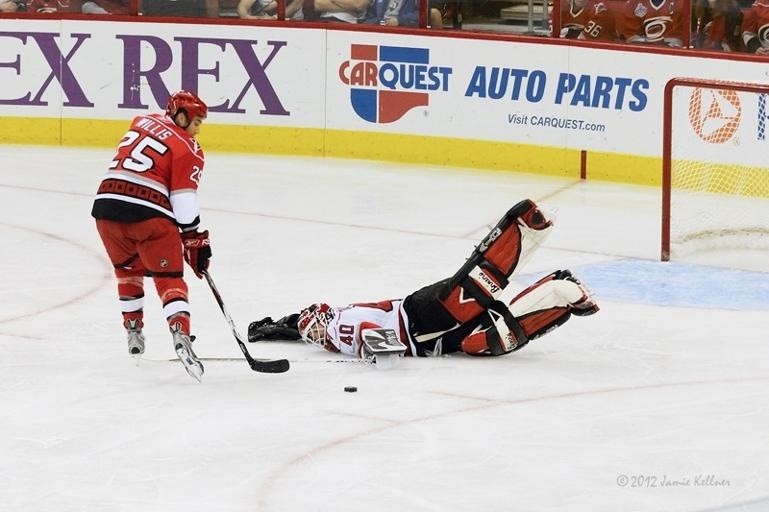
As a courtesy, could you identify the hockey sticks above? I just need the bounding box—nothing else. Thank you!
[168,358,368,362]
[202,269,289,373]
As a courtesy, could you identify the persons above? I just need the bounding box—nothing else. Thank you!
[93,92,205,378]
[0,2,769,55]
[295,198,599,358]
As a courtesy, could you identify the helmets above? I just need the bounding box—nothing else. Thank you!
[165,89,208,130]
[296,302,337,352]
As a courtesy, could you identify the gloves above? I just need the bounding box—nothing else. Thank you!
[179,227,212,280]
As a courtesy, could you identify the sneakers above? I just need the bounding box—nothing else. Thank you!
[127,331,145,355]
[173,331,204,377]
